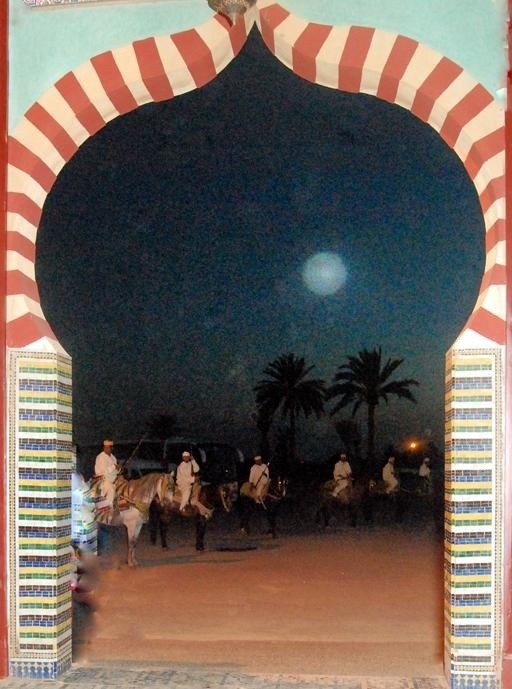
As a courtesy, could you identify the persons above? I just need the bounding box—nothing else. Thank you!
[175,449,201,513]
[94,437,124,524]
[381,457,397,495]
[330,453,354,498]
[417,457,434,495]
[248,454,271,506]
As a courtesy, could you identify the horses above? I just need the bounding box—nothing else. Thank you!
[313,472,375,534]
[148,482,233,554]
[85,470,175,567]
[376,480,412,527]
[238,475,290,540]
[396,479,444,524]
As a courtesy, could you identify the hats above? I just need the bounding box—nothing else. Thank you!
[181,451,191,457]
[388,457,395,461]
[253,455,262,461]
[424,458,430,462]
[102,438,115,446]
[340,454,346,457]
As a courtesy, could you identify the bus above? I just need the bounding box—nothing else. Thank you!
[76,436,245,503]
